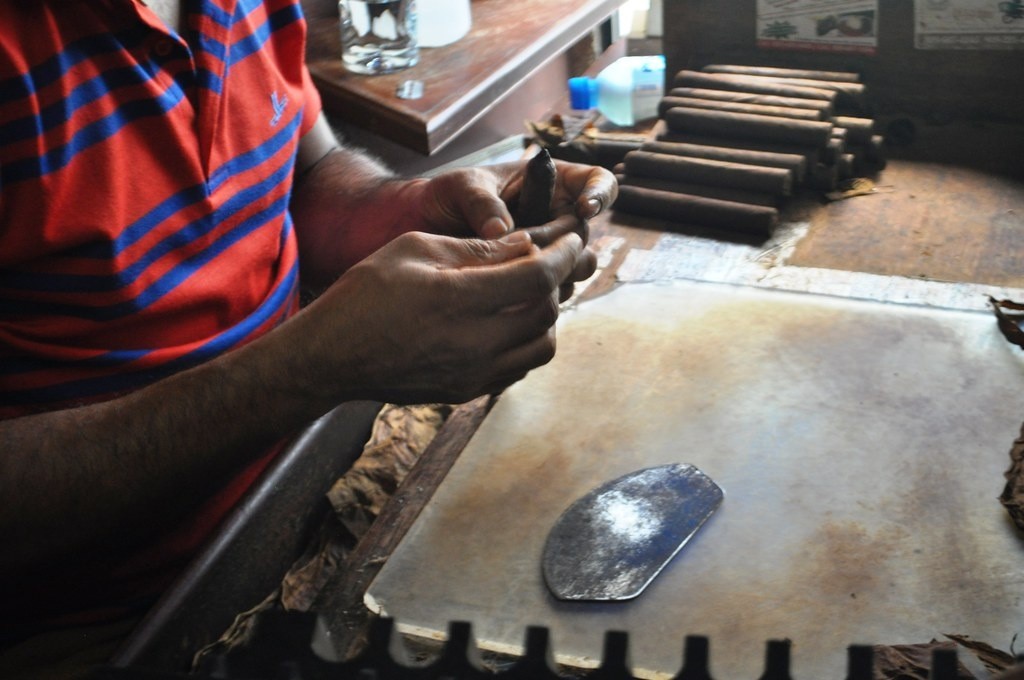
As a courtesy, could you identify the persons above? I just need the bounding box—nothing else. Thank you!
[0,1,618,680]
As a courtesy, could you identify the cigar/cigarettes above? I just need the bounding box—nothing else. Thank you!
[516,148,557,228]
[611,65,887,235]
[596,140,642,162]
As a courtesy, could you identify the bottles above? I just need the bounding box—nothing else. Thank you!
[568,55,667,126]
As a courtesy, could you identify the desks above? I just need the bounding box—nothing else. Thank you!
[300,0,629,183]
[113,35,1024,680]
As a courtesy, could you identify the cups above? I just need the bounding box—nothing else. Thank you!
[340,0,419,75]
